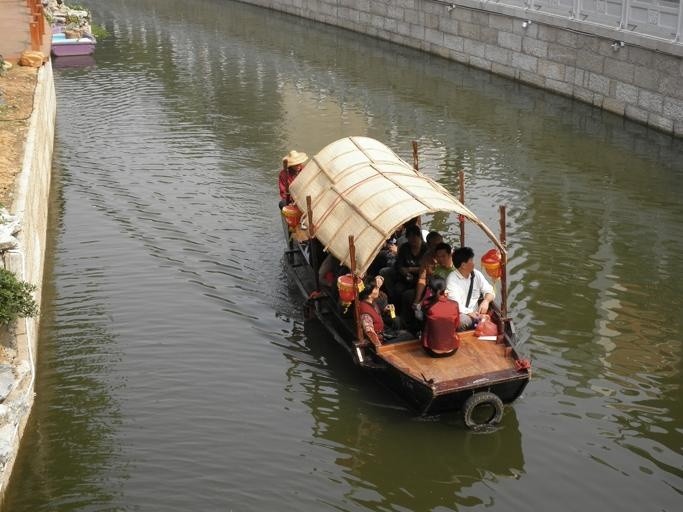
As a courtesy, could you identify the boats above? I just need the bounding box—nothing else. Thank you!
[40,1,98,57]
[278,138,534,433]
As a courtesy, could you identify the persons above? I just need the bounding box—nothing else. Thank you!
[354,217,496,349]
[279,150,308,210]
